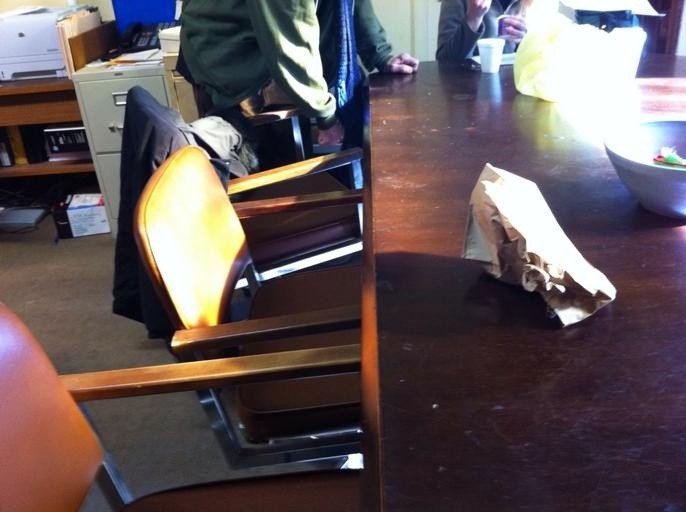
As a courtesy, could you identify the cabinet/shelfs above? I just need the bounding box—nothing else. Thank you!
[0,20,199,239]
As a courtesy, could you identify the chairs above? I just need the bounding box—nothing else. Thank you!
[0,297,367,510]
[134,147,366,468]
[221,148,364,281]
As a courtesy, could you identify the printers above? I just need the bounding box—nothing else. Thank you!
[0,5,103,82]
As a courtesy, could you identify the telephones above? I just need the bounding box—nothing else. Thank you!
[119,20,179,53]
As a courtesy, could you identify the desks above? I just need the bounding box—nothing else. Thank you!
[362,56,686,512]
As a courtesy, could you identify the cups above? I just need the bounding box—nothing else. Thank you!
[477,38,506,75]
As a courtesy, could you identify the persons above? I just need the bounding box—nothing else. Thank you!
[175,0,419,147]
[436,0,533,61]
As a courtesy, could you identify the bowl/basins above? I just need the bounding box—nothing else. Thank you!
[602,119,686,222]
